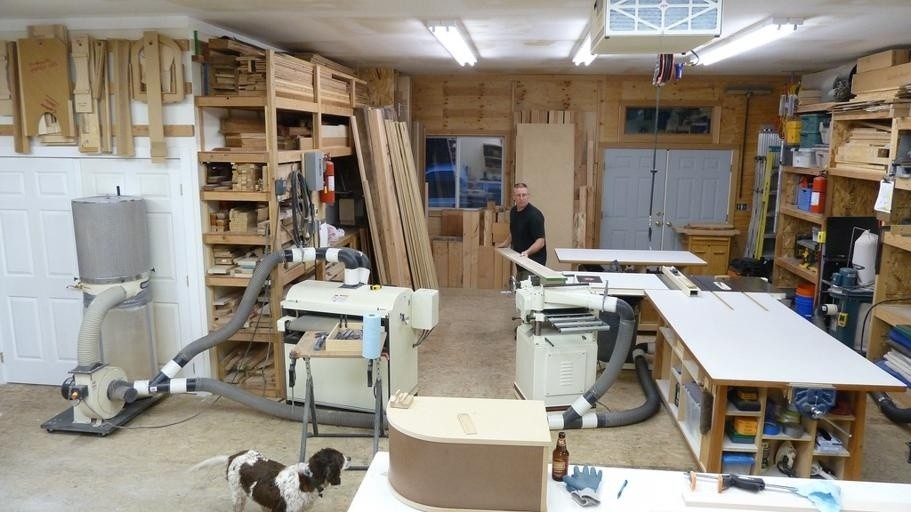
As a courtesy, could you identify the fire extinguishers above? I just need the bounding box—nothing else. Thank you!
[810,170,827,214]
[318,153,335,204]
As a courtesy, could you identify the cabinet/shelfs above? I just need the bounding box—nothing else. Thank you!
[760,145,785,261]
[768,106,911,412]
[641,281,909,484]
[194,54,386,405]
[679,232,734,276]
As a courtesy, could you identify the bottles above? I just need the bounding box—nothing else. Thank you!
[551,432,571,481]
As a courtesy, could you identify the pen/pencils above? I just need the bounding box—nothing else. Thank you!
[617,480,627,497]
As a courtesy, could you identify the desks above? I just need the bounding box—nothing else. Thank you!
[345,449,911,511]
[559,268,789,374]
[553,245,712,276]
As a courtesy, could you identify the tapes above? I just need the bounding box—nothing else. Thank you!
[764,420,780,435]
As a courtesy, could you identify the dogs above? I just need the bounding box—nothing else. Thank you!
[223,446,353,512]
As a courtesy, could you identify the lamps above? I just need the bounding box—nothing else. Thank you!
[566,20,604,70]
[685,12,808,71]
[417,15,483,69]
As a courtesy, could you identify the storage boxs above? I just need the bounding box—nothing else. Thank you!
[850,61,911,96]
[783,110,836,171]
[856,47,911,74]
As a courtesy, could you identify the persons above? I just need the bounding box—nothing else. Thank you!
[496,182,547,339]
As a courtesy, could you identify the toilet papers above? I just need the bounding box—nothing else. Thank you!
[362,314,381,360]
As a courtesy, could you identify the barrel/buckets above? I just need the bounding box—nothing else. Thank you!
[794,283,815,318]
